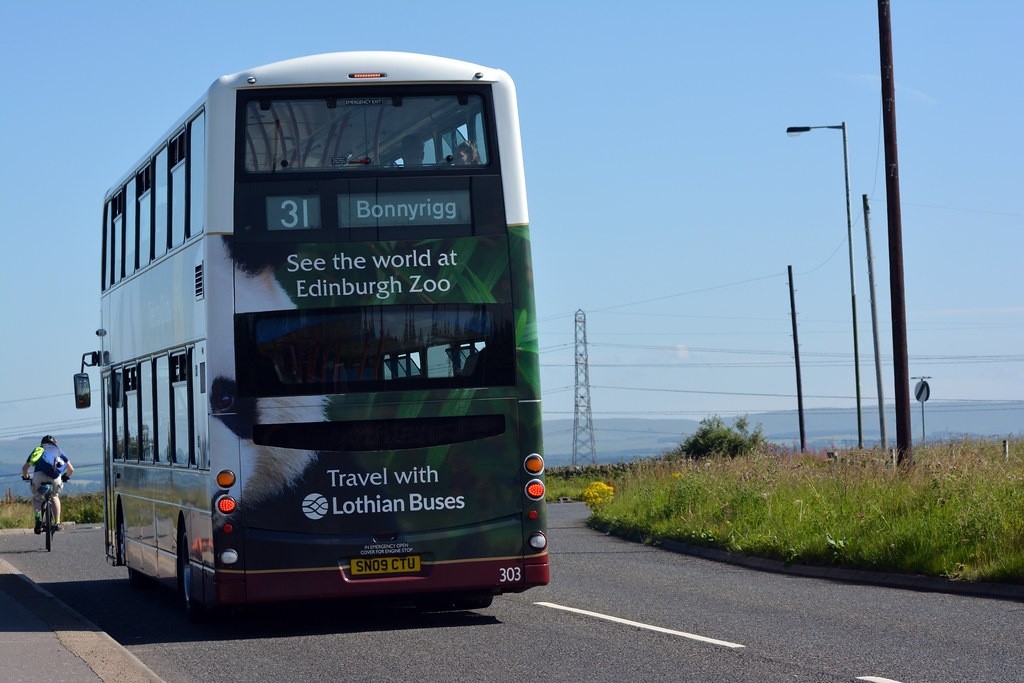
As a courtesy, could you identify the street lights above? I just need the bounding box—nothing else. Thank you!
[786,121,865,450]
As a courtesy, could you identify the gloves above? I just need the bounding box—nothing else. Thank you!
[61,473,69,482]
[22,475,30,480]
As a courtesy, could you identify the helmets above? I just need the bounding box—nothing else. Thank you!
[41,435,57,444]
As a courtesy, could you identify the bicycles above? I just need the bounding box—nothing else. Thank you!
[22,477,68,553]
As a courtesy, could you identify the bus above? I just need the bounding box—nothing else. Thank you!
[72,49,553,620]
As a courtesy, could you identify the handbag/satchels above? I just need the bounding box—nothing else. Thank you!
[29,447,44,467]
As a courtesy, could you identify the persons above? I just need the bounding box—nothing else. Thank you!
[19,434,74,535]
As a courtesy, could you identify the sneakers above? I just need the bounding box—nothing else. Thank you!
[54,521,64,531]
[34,519,43,535]
